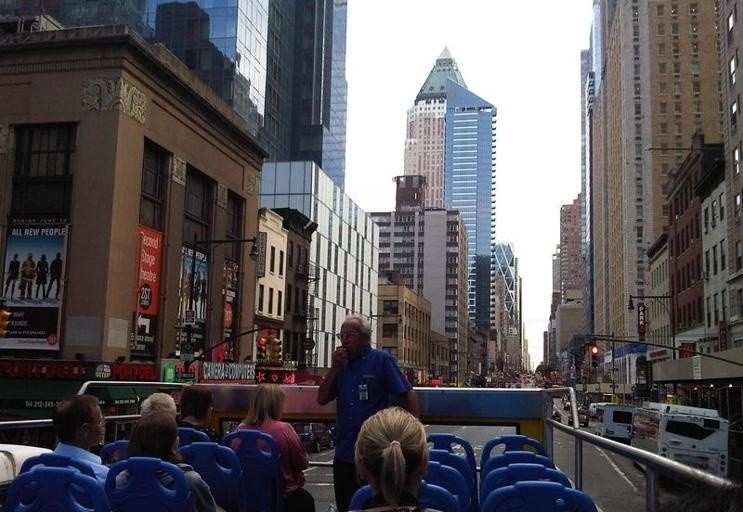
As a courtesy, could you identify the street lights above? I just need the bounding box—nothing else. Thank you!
[182,231,258,384]
[369,309,404,345]
[627,283,678,405]
[583,330,615,402]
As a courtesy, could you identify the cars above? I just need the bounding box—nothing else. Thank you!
[288,422,334,452]
[414,367,587,425]
[568,408,590,427]
[588,403,596,421]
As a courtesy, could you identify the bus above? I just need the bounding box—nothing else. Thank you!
[594,403,637,440]
[629,401,729,485]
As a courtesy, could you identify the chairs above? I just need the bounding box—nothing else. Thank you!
[346,431,597,512]
[1,428,285,511]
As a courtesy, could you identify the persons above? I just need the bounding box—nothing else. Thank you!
[231,383,315,511]
[115,412,218,512]
[4,252,62,300]
[177,385,222,444]
[193,271,206,319]
[30,395,112,488]
[139,393,176,420]
[317,313,419,512]
[346,406,443,512]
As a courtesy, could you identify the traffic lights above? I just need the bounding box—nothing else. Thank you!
[270,335,281,364]
[256,336,267,362]
[591,345,599,369]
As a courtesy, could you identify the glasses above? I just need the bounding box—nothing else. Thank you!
[336,332,356,340]
